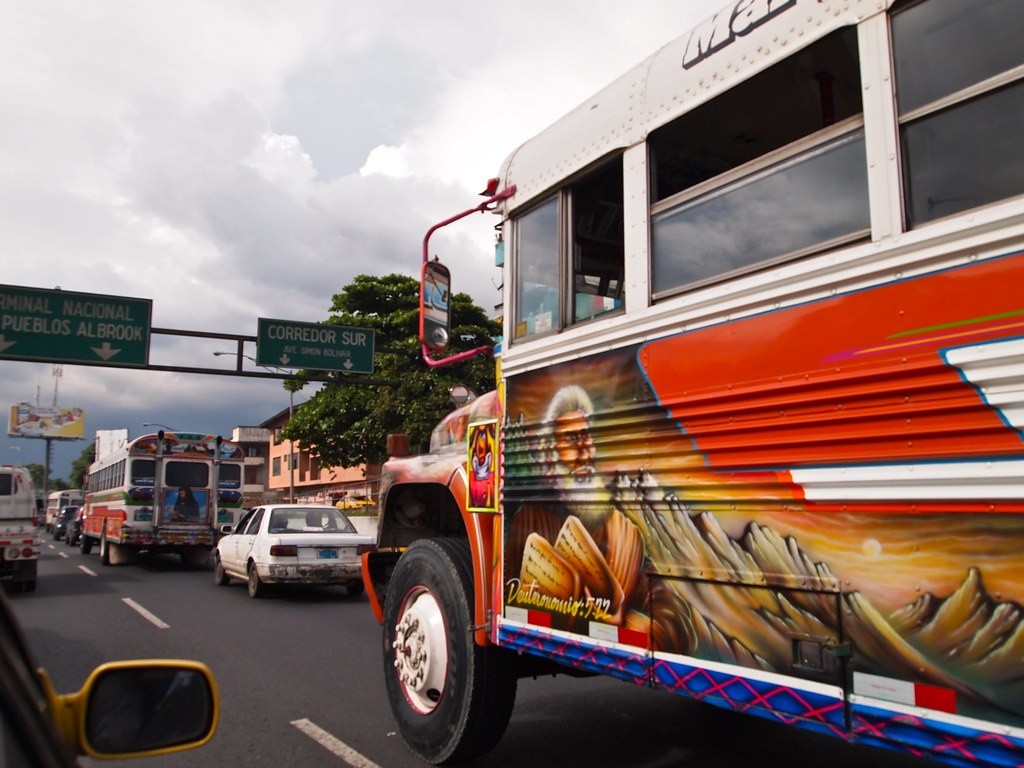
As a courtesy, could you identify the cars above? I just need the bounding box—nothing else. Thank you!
[212,502,376,598]
[335,492,376,508]
[52,505,82,541]
[65,506,86,546]
[1,587,222,768]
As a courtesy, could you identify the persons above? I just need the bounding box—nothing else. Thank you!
[90,676,142,753]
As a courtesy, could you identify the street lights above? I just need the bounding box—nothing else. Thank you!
[213,350,295,538]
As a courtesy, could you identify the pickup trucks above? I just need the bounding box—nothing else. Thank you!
[0,465,40,590]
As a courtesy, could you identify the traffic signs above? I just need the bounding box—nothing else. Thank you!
[0,284,153,369]
[257,316,373,370]
[363,3,1023,767]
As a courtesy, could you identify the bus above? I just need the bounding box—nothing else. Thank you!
[45,489,86,534]
[78,430,244,569]
